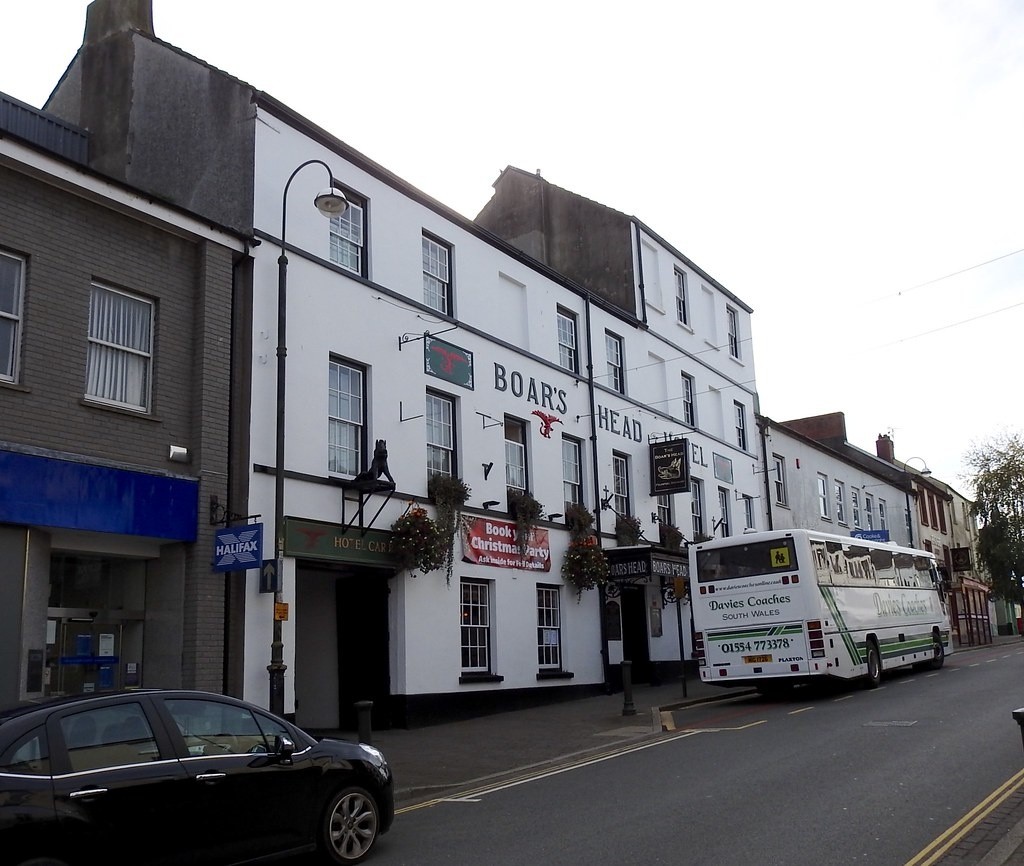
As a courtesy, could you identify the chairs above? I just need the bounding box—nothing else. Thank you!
[70,716,102,768]
[102,717,154,765]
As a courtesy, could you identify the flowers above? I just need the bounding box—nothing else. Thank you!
[561,544,611,603]
[389,508,452,578]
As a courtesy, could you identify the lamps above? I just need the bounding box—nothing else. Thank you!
[483,501,501,510]
[549,513,564,522]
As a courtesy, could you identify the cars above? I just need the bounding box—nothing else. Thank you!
[40,643,101,694]
[0,687,394,866]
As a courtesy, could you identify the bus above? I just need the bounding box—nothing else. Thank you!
[688,528,954,693]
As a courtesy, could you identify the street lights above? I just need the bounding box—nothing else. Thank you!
[268,160,349,717]
[904,457,932,549]
